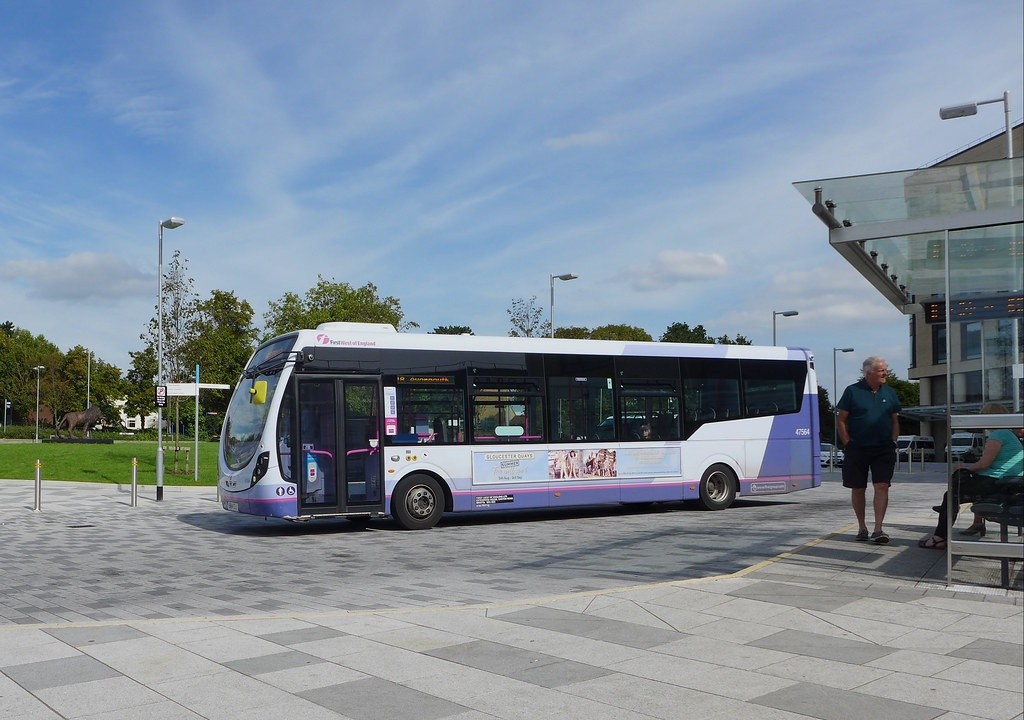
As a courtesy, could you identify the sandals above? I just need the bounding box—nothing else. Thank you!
[918,537,945,549]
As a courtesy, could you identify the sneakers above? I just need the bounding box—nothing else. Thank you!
[870,531,889,543]
[856,527,869,542]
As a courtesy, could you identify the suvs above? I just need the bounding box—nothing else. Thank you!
[592,410,679,441]
[820,441,845,467]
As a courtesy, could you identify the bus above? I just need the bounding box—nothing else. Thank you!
[218,321,820,530]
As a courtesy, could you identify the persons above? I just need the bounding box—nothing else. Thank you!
[835,356,902,544]
[918,402,1024,550]
[641,424,652,440]
[284,395,314,444]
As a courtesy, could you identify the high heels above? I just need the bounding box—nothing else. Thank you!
[932,506,941,512]
[959,523,986,536]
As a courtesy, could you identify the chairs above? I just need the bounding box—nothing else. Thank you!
[397,400,778,441]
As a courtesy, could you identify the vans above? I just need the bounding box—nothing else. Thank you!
[895,435,936,462]
[943,432,985,462]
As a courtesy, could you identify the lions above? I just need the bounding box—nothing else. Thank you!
[54,406,104,439]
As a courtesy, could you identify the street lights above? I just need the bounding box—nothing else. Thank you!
[33,366,44,443]
[772,311,799,346]
[158,217,186,502]
[550,273,579,337]
[83,348,91,437]
[833,348,854,467]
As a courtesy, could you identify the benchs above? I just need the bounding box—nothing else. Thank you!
[961,450,1024,591]
